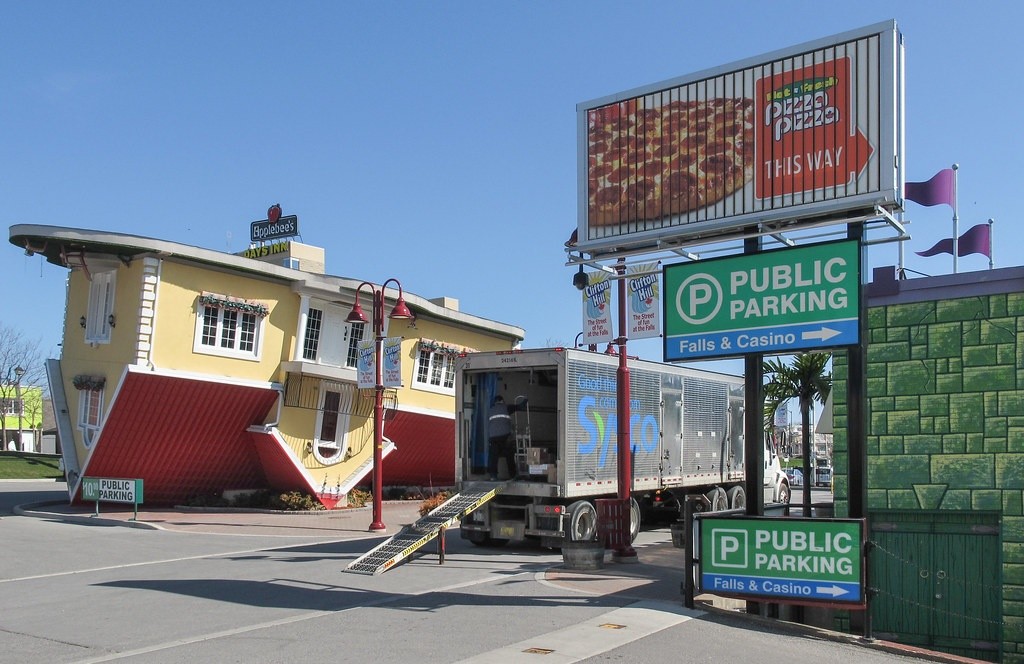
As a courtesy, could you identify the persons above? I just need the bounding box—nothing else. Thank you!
[488,396,528,482]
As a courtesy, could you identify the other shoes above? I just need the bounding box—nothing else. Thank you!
[490,474,497,481]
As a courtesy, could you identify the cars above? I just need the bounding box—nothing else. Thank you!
[782,468,794,483]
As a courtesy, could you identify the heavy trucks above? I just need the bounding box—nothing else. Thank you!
[449,348,791,549]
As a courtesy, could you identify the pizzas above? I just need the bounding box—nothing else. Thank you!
[588,95,756,225]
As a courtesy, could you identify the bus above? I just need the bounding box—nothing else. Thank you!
[794,467,832,487]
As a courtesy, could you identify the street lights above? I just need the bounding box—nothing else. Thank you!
[14,365,25,452]
[344,279,417,531]
